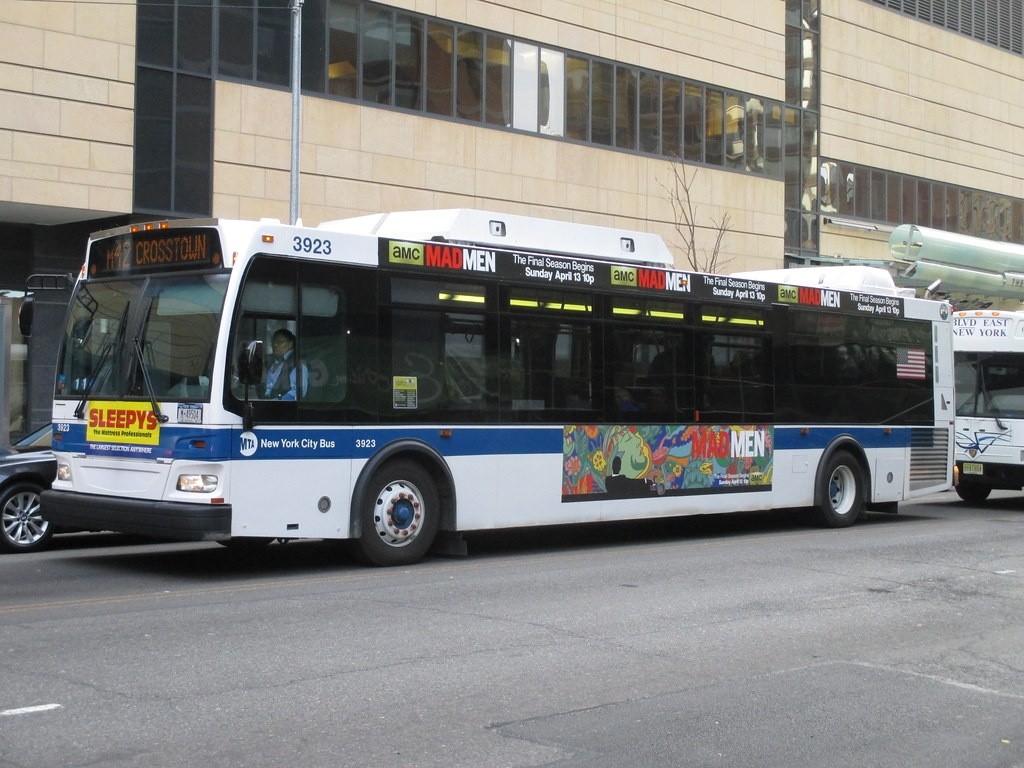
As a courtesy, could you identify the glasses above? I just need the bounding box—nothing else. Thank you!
[268,339,289,346]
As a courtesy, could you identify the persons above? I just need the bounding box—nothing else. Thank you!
[255,328,308,401]
[614,333,748,414]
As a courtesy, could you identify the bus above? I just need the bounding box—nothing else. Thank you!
[953,309,1024,504]
[38,210,955,569]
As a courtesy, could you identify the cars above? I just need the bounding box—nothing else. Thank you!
[0,421,90,554]
